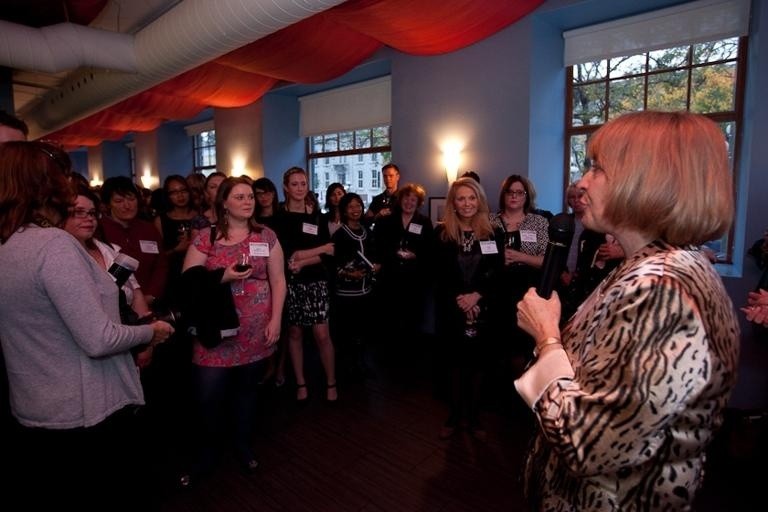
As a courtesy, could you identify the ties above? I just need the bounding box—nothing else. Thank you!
[564,219,585,275]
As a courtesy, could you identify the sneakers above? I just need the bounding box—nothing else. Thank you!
[471,429,487,442]
[437,425,457,439]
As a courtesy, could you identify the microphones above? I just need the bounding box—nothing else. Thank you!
[536,212,576,300]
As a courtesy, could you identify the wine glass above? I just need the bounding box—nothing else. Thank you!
[233,251,253,297]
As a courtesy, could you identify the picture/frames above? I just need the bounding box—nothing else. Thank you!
[427,195,448,230]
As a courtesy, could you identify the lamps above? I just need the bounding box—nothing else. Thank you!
[440,155,461,186]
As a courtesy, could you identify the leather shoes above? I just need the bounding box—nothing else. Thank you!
[295,383,310,407]
[323,382,340,407]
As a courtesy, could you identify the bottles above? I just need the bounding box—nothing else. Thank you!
[108,254,139,288]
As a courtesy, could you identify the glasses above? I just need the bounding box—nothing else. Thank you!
[503,189,529,196]
[69,207,106,221]
[576,158,607,175]
[256,189,273,198]
[166,187,188,197]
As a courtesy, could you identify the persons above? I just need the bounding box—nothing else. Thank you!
[511,109,741,511]
[740,284,768,327]
[742,229,768,424]
[1,139,174,512]
[1,106,25,148]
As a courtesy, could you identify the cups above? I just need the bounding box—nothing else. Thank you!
[397,240,408,264]
[505,235,514,250]
[466,312,478,336]
